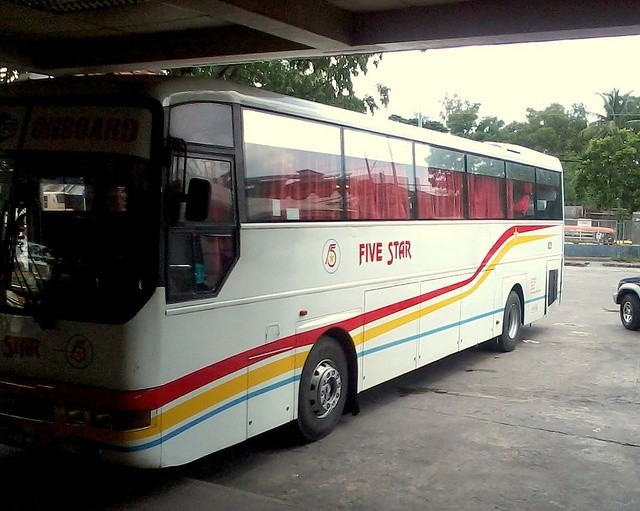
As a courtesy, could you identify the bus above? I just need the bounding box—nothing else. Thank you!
[1,77,564,468]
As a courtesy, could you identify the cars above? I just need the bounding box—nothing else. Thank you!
[614,277,640,330]
